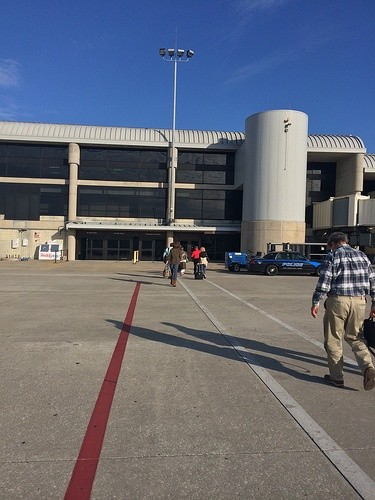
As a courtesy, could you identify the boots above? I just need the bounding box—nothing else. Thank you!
[171,279,173,284]
[173,279,176,286]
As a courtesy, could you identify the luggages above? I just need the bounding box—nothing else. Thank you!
[196,264,203,279]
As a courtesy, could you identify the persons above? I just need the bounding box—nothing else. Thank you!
[163,243,188,277]
[311,232,375,390]
[191,246,201,274]
[200,246,208,279]
[168,242,182,287]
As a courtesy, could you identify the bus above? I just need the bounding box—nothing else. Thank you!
[266,242,335,264]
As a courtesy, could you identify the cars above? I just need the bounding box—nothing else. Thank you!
[247,250,322,277]
[224,251,255,273]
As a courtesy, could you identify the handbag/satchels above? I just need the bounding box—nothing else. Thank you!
[163,264,170,276]
[364,317,375,348]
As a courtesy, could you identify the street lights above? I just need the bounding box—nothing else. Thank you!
[158,46,196,225]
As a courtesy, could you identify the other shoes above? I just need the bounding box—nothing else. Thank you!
[364,367,375,390]
[324,375,344,387]
